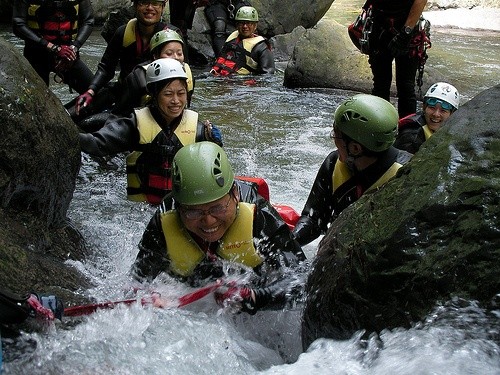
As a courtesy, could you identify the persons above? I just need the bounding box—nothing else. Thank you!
[62,0,185,109]
[68,29,195,133]
[203,0,253,60]
[168,0,215,65]
[395,82,461,157]
[366,0,427,119]
[208,7,276,75]
[292,94,416,259]
[123,141,308,318]
[12,0,95,94]
[71,58,222,203]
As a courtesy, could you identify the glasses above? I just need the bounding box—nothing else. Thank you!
[238,21,255,26]
[139,1,162,8]
[180,194,235,221]
[425,98,452,111]
[330,130,346,141]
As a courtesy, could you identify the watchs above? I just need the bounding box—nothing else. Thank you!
[403,25,413,35]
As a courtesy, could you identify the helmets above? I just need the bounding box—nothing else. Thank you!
[146,58,188,91]
[335,93,399,152]
[150,30,185,53]
[424,81,459,110]
[235,6,259,22]
[171,141,236,206]
